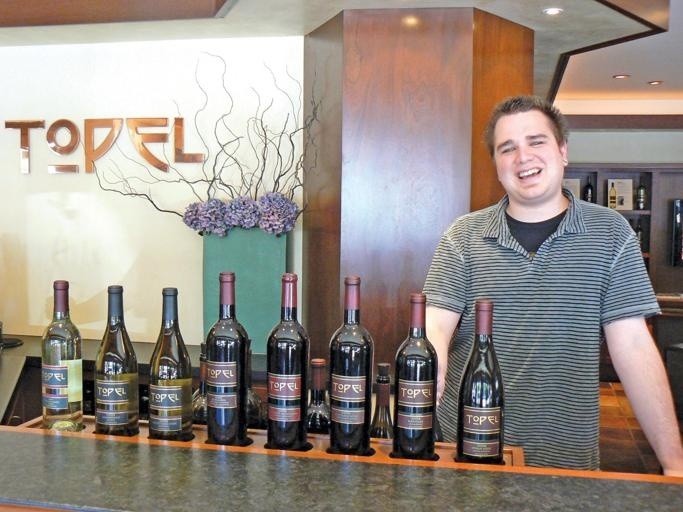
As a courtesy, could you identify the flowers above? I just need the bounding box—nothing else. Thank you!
[180,192,295,239]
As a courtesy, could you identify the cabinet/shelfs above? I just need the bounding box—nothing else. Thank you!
[558,162,661,289]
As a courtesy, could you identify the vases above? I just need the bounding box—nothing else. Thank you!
[201,225,287,353]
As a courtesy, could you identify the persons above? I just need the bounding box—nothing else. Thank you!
[411,95,683,481]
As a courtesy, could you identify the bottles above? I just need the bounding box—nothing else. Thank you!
[636,176,647,209]
[148,287,193,441]
[265,271,309,450]
[582,176,593,202]
[456,299,505,464]
[628,218,634,230]
[327,275,375,454]
[392,293,438,461]
[608,183,617,209]
[95,284,141,437]
[192,335,446,442]
[204,271,249,447]
[38,280,85,435]
[636,219,644,252]
